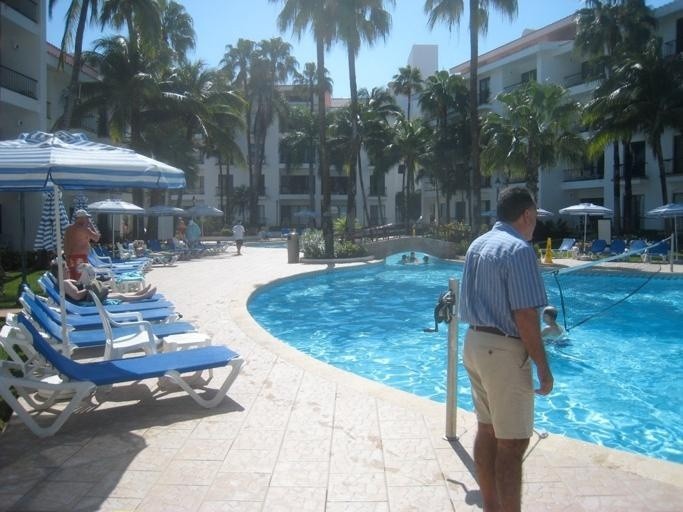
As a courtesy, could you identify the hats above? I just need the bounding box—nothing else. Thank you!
[75,210,91,217]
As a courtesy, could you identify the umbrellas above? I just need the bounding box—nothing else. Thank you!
[559,202,614,254]
[87,198,144,257]
[644,202,683,259]
[184,205,224,248]
[0,130,186,382]
[534,208,555,243]
[33,190,70,254]
[137,204,184,215]
[294,210,321,227]
[71,190,102,239]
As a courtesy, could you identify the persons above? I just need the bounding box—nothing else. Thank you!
[397,252,428,264]
[64,209,100,280]
[133,240,177,265]
[539,307,563,338]
[51,257,157,302]
[459,186,554,512]
[233,221,244,255]
[123,215,129,249]
[178,217,200,249]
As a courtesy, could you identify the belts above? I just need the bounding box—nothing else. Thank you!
[469,325,522,339]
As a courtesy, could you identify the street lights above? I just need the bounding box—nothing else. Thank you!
[495,176,500,201]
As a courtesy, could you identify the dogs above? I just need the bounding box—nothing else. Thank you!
[75,261,98,288]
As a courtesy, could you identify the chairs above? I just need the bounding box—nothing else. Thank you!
[550,236,668,263]
[280,228,291,241]
